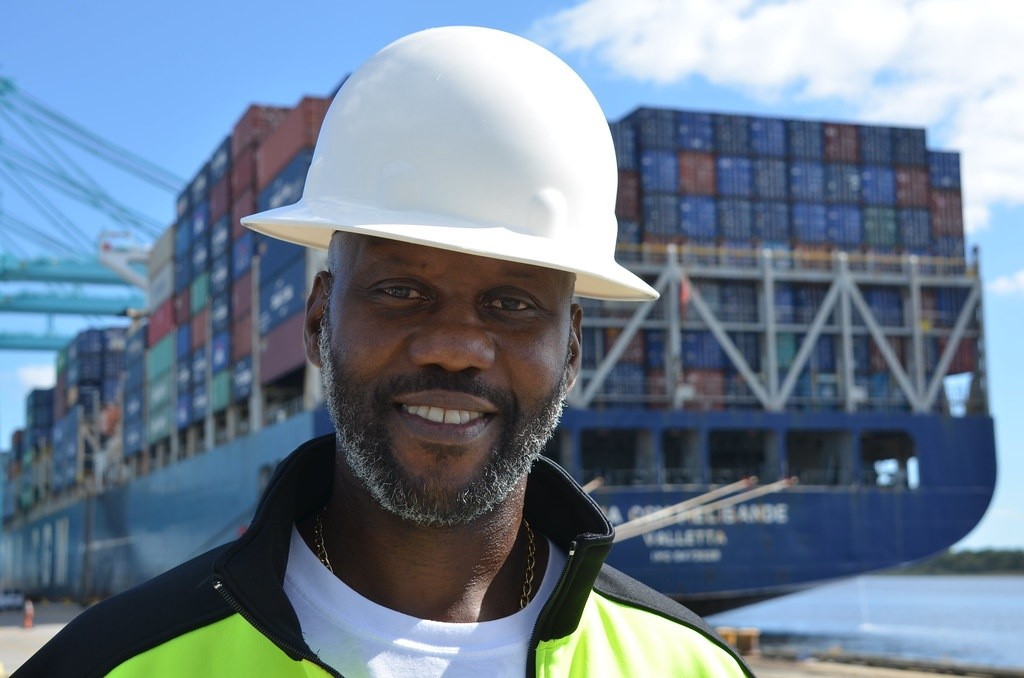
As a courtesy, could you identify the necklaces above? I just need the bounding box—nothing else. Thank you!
[314,494,535,613]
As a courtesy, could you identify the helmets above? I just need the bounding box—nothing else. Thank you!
[239,25,660,301]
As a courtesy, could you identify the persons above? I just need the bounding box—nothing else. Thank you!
[9,26,760,678]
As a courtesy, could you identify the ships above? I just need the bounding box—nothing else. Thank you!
[2,222,998,618]
